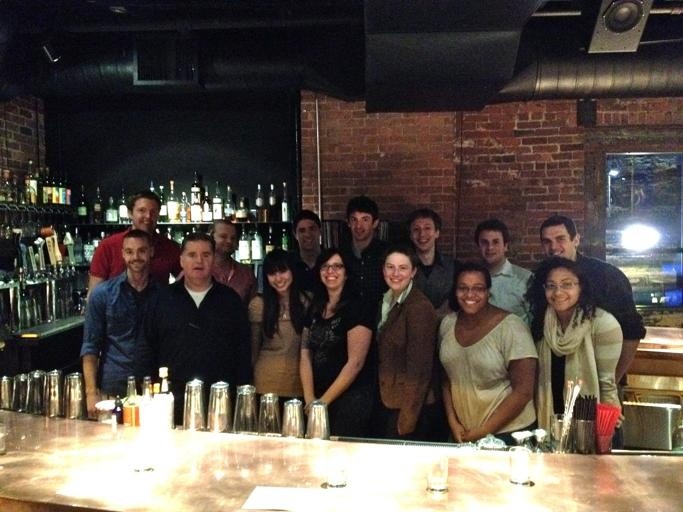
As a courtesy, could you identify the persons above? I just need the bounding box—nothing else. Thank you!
[287,207,328,312]
[299,247,373,439]
[403,208,460,327]
[340,197,394,325]
[150,232,253,426]
[436,262,538,447]
[86,194,185,306]
[529,257,627,451]
[435,216,536,337]
[247,248,315,430]
[79,229,171,421]
[174,219,261,305]
[367,248,439,441]
[527,214,646,445]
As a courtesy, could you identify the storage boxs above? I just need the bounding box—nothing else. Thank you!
[621,402,680,451]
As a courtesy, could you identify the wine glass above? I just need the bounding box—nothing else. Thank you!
[507,427,548,485]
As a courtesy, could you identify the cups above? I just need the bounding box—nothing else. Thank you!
[206,381,230,432]
[455,434,507,479]
[305,400,331,440]
[0,370,43,417]
[423,455,449,491]
[42,369,61,419]
[63,373,84,419]
[549,414,572,455]
[594,434,614,454]
[572,419,597,456]
[182,379,206,431]
[281,399,305,438]
[257,393,280,434]
[232,383,256,433]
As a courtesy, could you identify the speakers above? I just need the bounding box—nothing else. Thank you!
[580,0,653,54]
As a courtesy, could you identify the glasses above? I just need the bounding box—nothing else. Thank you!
[542,280,578,289]
[321,263,343,271]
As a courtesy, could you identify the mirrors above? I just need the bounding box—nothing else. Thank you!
[580,125,683,360]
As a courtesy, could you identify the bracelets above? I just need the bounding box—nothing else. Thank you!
[84,387,101,397]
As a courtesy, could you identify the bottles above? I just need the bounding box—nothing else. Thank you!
[139,366,174,430]
[124,376,138,430]
[0,156,73,206]
[114,394,123,426]
[0,219,88,336]
[82,230,291,263]
[78,168,295,226]
